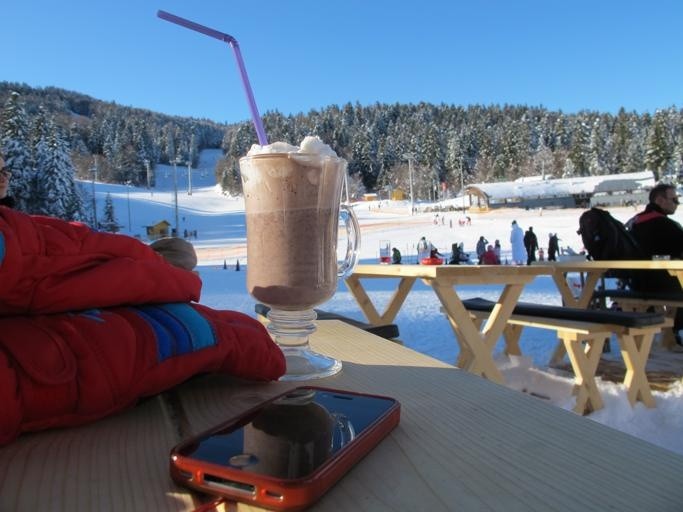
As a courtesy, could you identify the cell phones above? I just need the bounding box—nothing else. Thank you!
[171,385,401,512]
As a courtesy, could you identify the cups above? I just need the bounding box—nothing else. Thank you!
[378,239,392,264]
[417,240,430,263]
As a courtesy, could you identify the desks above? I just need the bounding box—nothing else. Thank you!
[1,318,682,511]
[338,266,555,387]
[534,259,683,367]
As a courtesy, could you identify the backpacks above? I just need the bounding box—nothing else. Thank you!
[576,206,665,279]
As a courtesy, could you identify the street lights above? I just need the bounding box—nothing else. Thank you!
[184,160,193,195]
[87,164,100,227]
[451,148,466,215]
[122,179,130,233]
[142,158,153,190]
[400,153,418,215]
[342,162,350,206]
[168,157,184,235]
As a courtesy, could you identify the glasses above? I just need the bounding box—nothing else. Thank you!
[667,197,680,205]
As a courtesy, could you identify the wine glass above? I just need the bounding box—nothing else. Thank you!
[233,153,361,378]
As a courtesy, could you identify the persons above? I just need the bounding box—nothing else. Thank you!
[494,239,502,265]
[509,219,526,266]
[619,183,683,349]
[391,247,402,263]
[0,153,15,210]
[448,242,459,264]
[416,236,426,264]
[483,244,498,265]
[538,248,544,260]
[547,233,557,261]
[553,233,561,257]
[476,237,488,263]
[429,248,445,258]
[457,243,471,261]
[561,246,586,255]
[522,226,539,267]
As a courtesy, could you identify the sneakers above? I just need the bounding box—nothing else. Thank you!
[661,334,683,353]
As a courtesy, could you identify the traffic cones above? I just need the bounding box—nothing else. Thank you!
[222,260,228,269]
[233,259,240,270]
